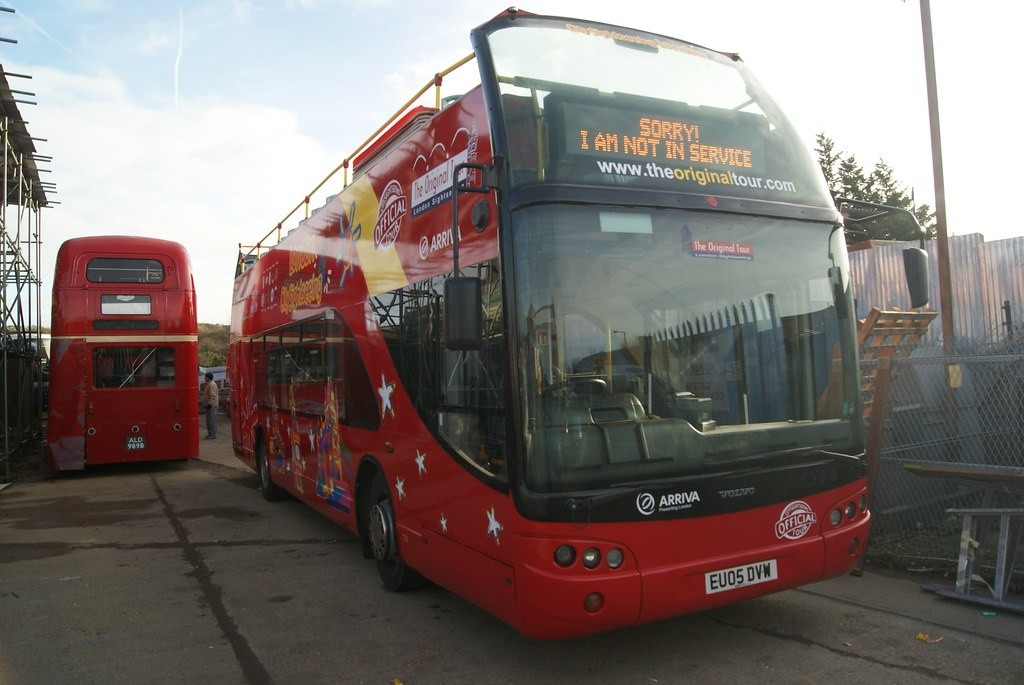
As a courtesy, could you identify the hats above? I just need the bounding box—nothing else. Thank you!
[205,372,213,380]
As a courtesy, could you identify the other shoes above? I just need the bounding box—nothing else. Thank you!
[205,433,216,439]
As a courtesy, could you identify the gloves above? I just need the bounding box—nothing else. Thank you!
[207,403,212,409]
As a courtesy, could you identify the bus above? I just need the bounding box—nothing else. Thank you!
[259,315,347,417]
[48,238,201,470]
[224,7,933,638]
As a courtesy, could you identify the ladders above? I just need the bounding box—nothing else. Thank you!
[921,507,1024,615]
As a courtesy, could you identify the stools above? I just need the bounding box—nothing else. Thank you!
[96,357,114,386]
[124,357,148,379]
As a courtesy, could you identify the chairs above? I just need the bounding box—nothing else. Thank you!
[243,193,338,272]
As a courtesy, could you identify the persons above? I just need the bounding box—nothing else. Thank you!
[199,373,219,439]
[524,307,599,396]
